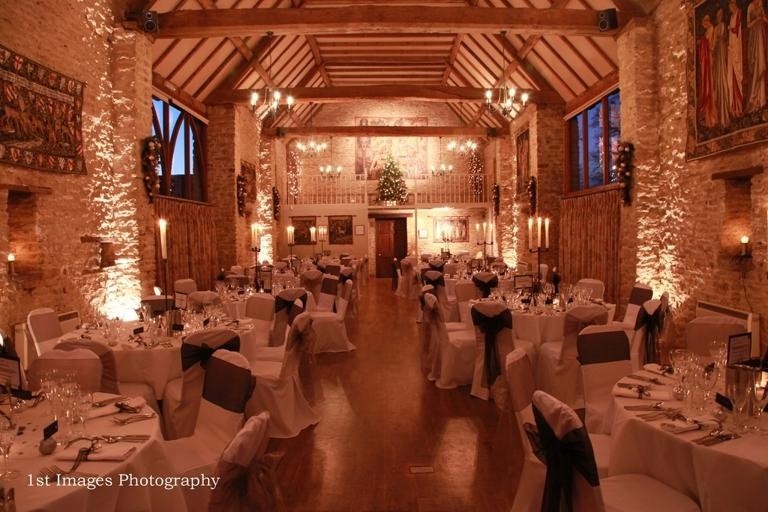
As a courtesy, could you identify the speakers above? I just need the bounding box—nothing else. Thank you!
[276,127,286,137]
[598,8,618,32]
[487,128,496,137]
[143,10,159,33]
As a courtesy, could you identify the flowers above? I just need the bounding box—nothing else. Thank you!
[611,141,636,208]
[492,182,500,215]
[525,177,538,210]
[270,185,283,218]
[238,175,249,214]
[139,130,164,203]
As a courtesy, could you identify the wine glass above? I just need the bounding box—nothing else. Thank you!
[217,277,295,300]
[490,282,594,316]
[444,266,514,281]
[38,366,93,445]
[669,340,768,441]
[75,301,228,348]
[0,419,20,480]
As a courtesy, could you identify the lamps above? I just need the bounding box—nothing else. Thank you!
[244,29,298,123]
[741,236,751,255]
[287,99,330,158]
[448,100,479,159]
[481,28,528,119]
[429,134,457,179]
[316,133,344,181]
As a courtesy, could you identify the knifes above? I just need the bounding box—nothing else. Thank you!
[90,396,127,408]
[691,428,743,446]
[98,434,150,443]
[0,486,17,511]
[614,373,669,422]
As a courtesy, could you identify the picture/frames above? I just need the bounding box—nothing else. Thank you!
[354,116,428,181]
[678,0,768,162]
[433,217,470,242]
[291,215,318,245]
[355,225,365,236]
[328,215,354,245]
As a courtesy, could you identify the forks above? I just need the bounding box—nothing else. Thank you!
[110,412,157,425]
[37,464,98,482]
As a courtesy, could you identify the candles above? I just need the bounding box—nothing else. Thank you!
[476,224,480,243]
[287,226,294,244]
[160,218,168,259]
[490,224,493,242]
[441,224,452,240]
[309,226,327,241]
[545,218,549,248]
[537,217,542,247]
[251,224,261,249]
[483,222,487,242]
[528,217,534,249]
